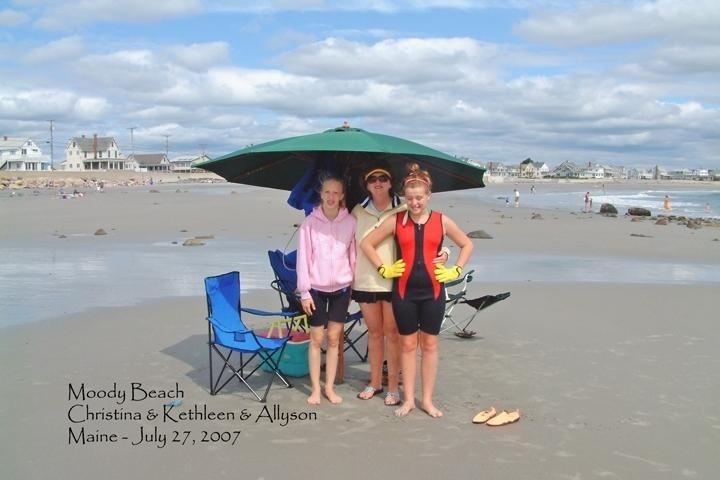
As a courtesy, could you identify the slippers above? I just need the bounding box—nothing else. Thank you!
[385,390,400,406]
[358,384,384,400]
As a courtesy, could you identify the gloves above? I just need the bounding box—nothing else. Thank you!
[434,263,463,282]
[376,259,406,279]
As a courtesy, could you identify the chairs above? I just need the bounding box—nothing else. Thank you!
[267,248,369,370]
[436,269,510,337]
[204,270,298,403]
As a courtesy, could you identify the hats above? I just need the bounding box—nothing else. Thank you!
[363,166,393,182]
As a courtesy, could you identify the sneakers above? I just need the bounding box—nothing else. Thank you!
[473,406,496,423]
[487,410,520,426]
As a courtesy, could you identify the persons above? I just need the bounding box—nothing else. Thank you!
[100,181,104,192]
[664,195,671,209]
[514,189,520,208]
[296,173,358,405]
[359,164,475,419]
[505,197,510,207]
[349,164,451,407]
[583,191,591,213]
[60,188,82,199]
[531,185,535,193]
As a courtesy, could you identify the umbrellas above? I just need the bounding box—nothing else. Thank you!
[187,120,486,383]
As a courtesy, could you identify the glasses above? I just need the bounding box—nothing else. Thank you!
[367,174,388,183]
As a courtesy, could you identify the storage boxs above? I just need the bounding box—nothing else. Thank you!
[250,326,309,377]
